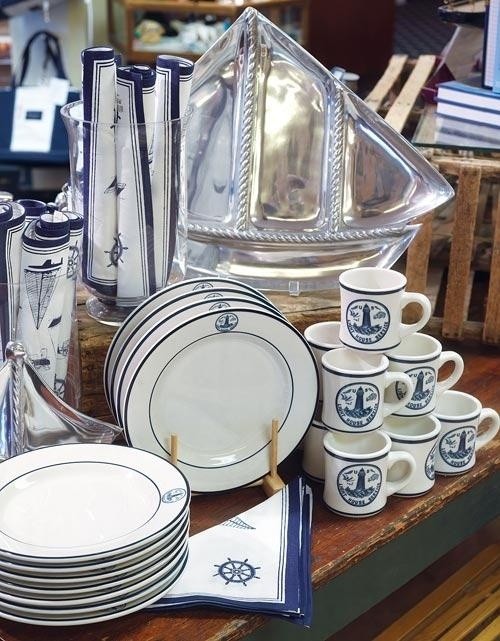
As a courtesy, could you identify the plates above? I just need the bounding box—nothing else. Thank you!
[0,441,191,628]
[101,276,320,497]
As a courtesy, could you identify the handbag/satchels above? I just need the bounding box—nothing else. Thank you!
[0,30,80,169]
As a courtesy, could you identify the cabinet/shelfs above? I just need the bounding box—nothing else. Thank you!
[124,2,311,69]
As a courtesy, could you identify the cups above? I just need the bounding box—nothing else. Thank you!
[382,414,441,500]
[303,322,345,406]
[0,270,80,454]
[322,430,417,520]
[384,331,465,420]
[322,348,415,438]
[336,268,431,353]
[431,391,500,479]
[302,418,329,485]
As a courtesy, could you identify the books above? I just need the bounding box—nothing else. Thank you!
[432,0,500,146]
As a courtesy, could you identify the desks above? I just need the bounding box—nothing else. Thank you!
[2,284,499,634]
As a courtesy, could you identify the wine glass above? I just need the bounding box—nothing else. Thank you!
[60,100,197,330]
